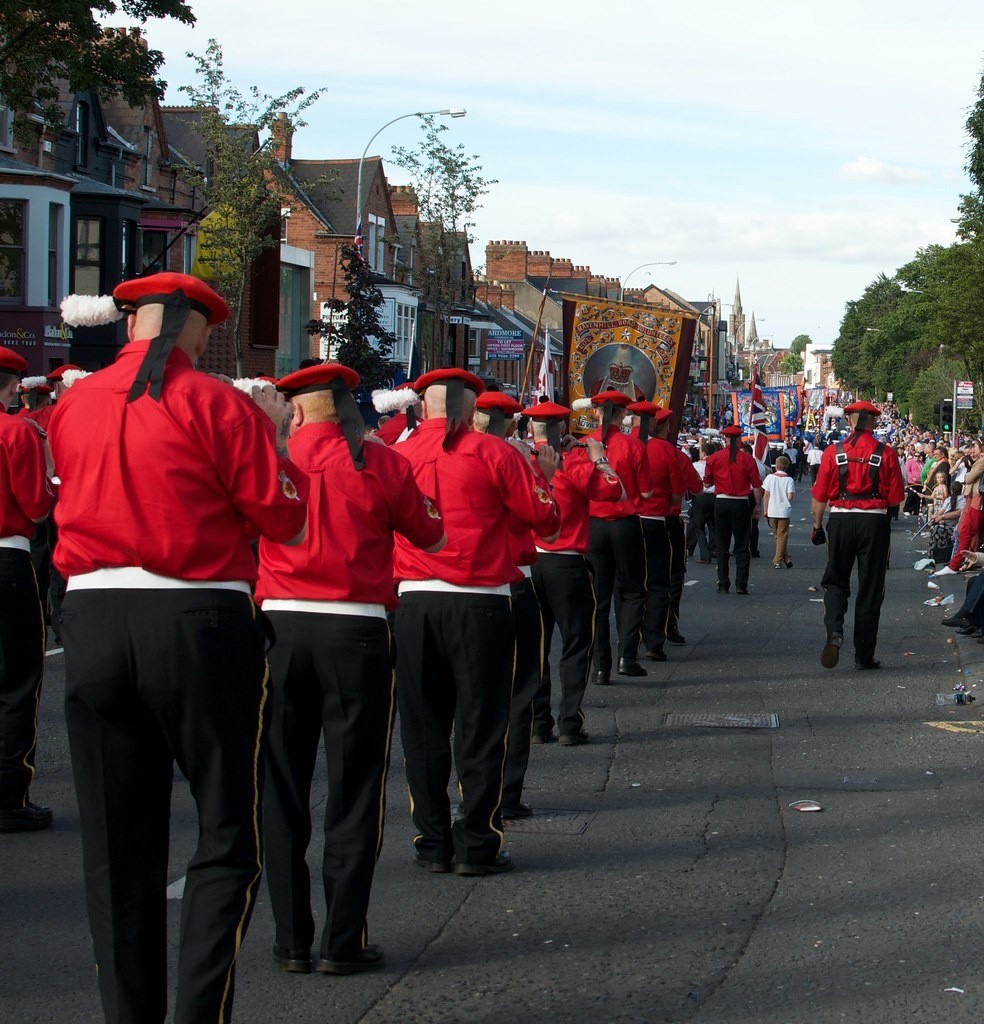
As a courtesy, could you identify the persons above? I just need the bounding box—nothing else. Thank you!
[793,400,984,643]
[811,402,905,671]
[0,349,794,976]
[46,271,312,1024]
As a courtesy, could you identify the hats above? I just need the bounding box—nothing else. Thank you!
[719,425,743,438]
[844,401,881,417]
[371,366,674,454]
[0,346,94,412]
[232,363,366,471]
[60,272,230,401]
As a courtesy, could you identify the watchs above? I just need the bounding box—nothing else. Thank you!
[595,456,608,466]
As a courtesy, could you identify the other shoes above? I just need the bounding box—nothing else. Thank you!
[854,657,882,670]
[774,563,783,569]
[786,560,793,569]
[820,632,844,669]
[715,586,729,595]
[736,586,748,595]
[0,801,53,833]
[688,549,760,564]
[941,612,984,645]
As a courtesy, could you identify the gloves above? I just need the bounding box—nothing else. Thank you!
[811,527,825,546]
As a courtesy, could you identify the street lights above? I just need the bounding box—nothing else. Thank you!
[356,109,466,349]
[780,362,793,383]
[695,304,734,383]
[620,260,677,306]
[749,334,774,381]
[735,318,765,378]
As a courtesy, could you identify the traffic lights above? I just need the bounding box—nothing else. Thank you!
[933,404,940,426]
[939,402,952,433]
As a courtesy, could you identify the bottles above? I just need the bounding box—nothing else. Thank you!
[935,693,975,706]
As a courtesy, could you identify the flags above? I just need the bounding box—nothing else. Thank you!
[747,367,771,468]
[354,219,364,259]
[531,332,555,406]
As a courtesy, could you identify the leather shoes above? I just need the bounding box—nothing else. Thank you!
[666,634,686,646]
[412,850,453,873]
[272,938,314,974]
[500,801,533,820]
[644,649,669,662]
[590,666,611,685]
[531,729,590,746]
[452,850,515,876]
[457,801,465,814]
[617,656,648,677]
[315,943,386,975]
